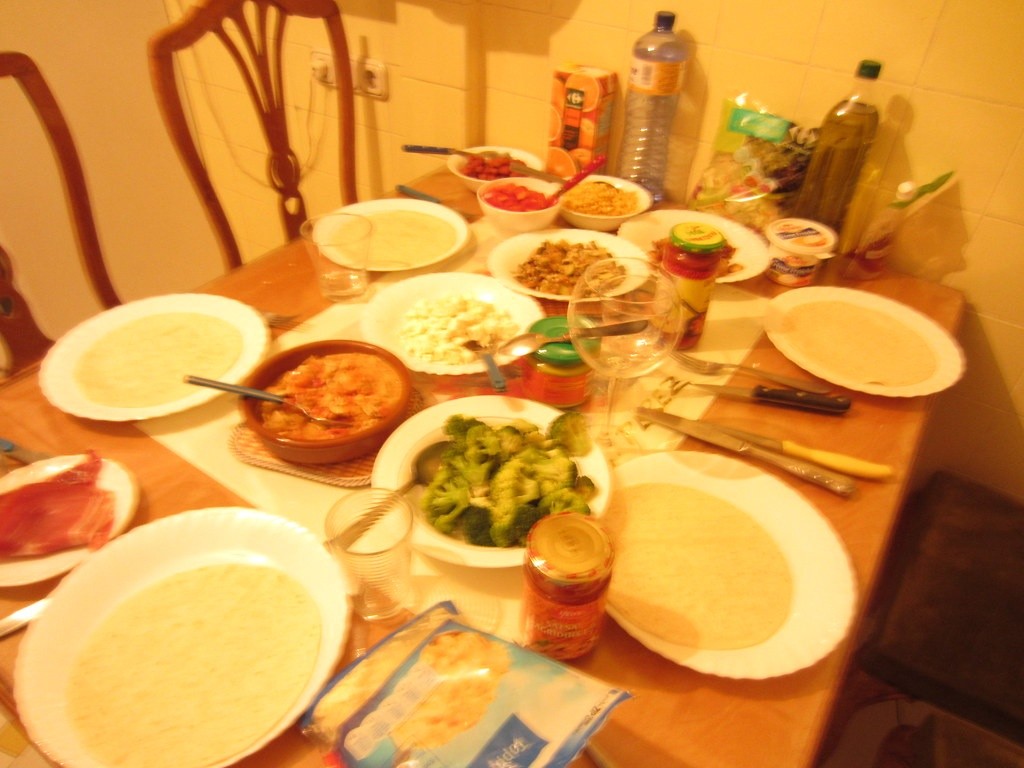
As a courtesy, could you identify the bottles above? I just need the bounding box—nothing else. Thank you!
[795,59,881,238]
[615,10,686,202]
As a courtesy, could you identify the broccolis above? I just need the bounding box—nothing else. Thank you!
[420,411,594,548]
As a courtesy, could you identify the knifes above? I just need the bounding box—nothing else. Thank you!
[633,406,895,497]
[0,597,48,642]
[397,185,480,223]
[690,384,852,412]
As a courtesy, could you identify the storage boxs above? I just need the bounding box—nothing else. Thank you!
[544,64,617,190]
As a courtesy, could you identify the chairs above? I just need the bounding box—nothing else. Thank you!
[858,464,1024,749]
[150,0,356,273]
[0,51,121,381]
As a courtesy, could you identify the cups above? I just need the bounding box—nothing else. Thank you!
[324,488,414,622]
[302,213,372,302]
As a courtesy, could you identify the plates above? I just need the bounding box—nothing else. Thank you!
[592,453,858,680]
[13,507,348,768]
[487,229,651,304]
[0,455,139,588]
[37,293,269,423]
[617,209,772,284]
[313,197,469,272]
[361,273,546,376]
[370,395,611,569]
[760,288,966,398]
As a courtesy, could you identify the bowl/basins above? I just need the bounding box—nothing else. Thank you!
[477,178,561,232]
[447,145,545,194]
[238,339,411,464]
[550,175,654,233]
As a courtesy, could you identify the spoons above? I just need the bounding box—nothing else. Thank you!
[183,376,353,427]
[497,320,650,357]
[323,440,455,558]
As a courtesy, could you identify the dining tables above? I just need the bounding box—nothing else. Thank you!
[0,152,966,768]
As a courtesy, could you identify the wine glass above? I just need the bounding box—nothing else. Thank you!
[566,258,684,472]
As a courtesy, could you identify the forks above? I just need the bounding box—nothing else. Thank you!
[257,311,301,326]
[664,343,833,395]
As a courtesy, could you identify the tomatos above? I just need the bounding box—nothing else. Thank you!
[461,156,527,179]
[480,182,551,212]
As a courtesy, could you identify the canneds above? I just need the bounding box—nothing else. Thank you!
[649,223,724,351]
[521,315,602,407]
[521,513,615,660]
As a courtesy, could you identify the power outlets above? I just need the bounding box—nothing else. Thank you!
[356,58,387,101]
[309,51,335,88]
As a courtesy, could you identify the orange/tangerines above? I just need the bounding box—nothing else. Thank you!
[547,75,601,181]
